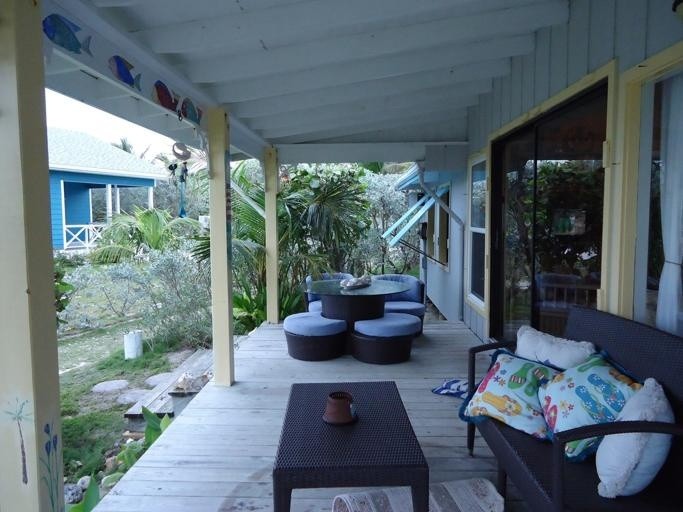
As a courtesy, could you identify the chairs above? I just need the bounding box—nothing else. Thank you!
[364,273,424,335]
[303,272,354,313]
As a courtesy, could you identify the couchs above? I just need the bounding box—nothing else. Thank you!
[461,305,683,512]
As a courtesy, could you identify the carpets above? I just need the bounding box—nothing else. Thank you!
[432,379,469,399]
[332,476,506,512]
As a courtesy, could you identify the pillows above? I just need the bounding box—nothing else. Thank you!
[458,348,564,444]
[514,325,594,372]
[596,378,675,498]
[537,350,644,461]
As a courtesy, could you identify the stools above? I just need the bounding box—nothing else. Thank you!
[283,311,347,361]
[353,313,422,364]
